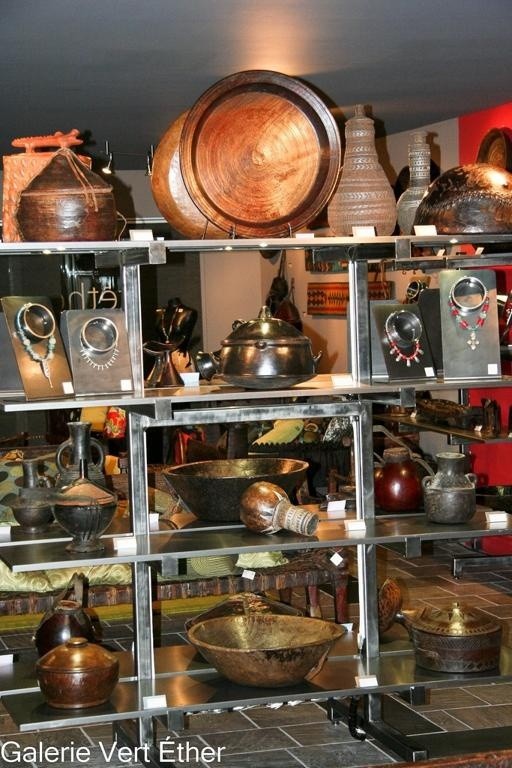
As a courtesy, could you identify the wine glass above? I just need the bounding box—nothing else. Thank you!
[377,575,428,643]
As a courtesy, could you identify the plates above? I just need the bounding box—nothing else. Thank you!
[150,108,231,241]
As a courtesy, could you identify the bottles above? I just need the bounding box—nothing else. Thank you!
[329,99,400,238]
[395,124,440,235]
[44,458,120,555]
[237,481,318,541]
[12,459,57,535]
[33,600,96,659]
[52,419,109,489]
[374,446,425,512]
[420,448,480,525]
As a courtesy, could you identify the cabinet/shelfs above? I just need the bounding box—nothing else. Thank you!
[0,233,512,767]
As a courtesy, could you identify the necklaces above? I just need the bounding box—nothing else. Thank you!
[79,329,119,370]
[17,303,57,388]
[385,311,424,367]
[448,276,489,350]
[160,304,182,344]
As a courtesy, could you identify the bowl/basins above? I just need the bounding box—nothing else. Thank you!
[14,147,123,243]
[474,484,512,513]
[34,637,121,712]
[185,613,349,690]
[159,455,312,525]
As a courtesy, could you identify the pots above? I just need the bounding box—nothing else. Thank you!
[408,599,503,674]
[212,305,325,389]
[183,592,305,649]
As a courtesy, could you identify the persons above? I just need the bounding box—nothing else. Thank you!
[158,297,198,354]
